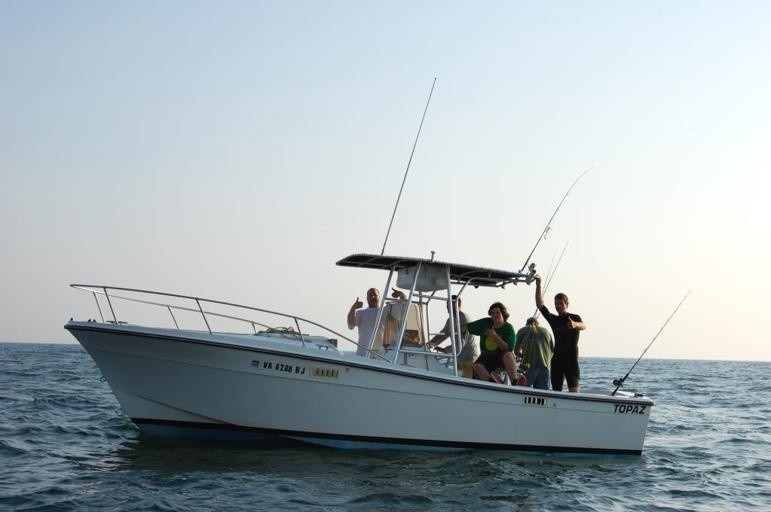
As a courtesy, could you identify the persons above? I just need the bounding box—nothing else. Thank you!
[347,287,407,360]
[513,317,553,391]
[464,303,525,386]
[425,296,478,379]
[535,275,587,392]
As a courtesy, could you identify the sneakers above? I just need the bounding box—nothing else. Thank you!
[511,375,526,385]
[488,371,502,383]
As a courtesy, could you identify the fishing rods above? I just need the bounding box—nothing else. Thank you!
[526,247,566,325]
[610,291,688,398]
[517,175,587,277]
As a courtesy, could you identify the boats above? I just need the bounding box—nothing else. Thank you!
[61,76,689,457]
